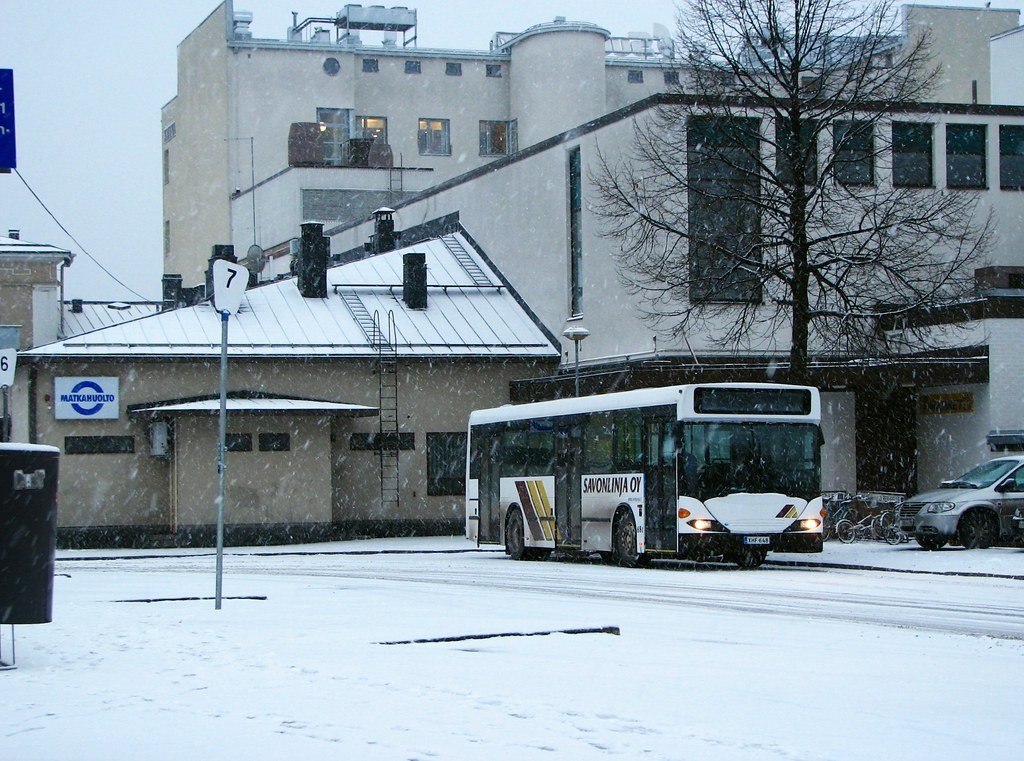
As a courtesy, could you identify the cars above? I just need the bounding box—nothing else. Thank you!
[898,456,1024,550]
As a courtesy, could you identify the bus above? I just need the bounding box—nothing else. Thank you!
[465,382,827,569]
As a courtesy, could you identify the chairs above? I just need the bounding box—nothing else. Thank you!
[708,456,741,491]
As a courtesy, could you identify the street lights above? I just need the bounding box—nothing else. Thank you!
[562,324,591,397]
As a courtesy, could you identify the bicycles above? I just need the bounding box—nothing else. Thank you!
[821,493,909,546]
